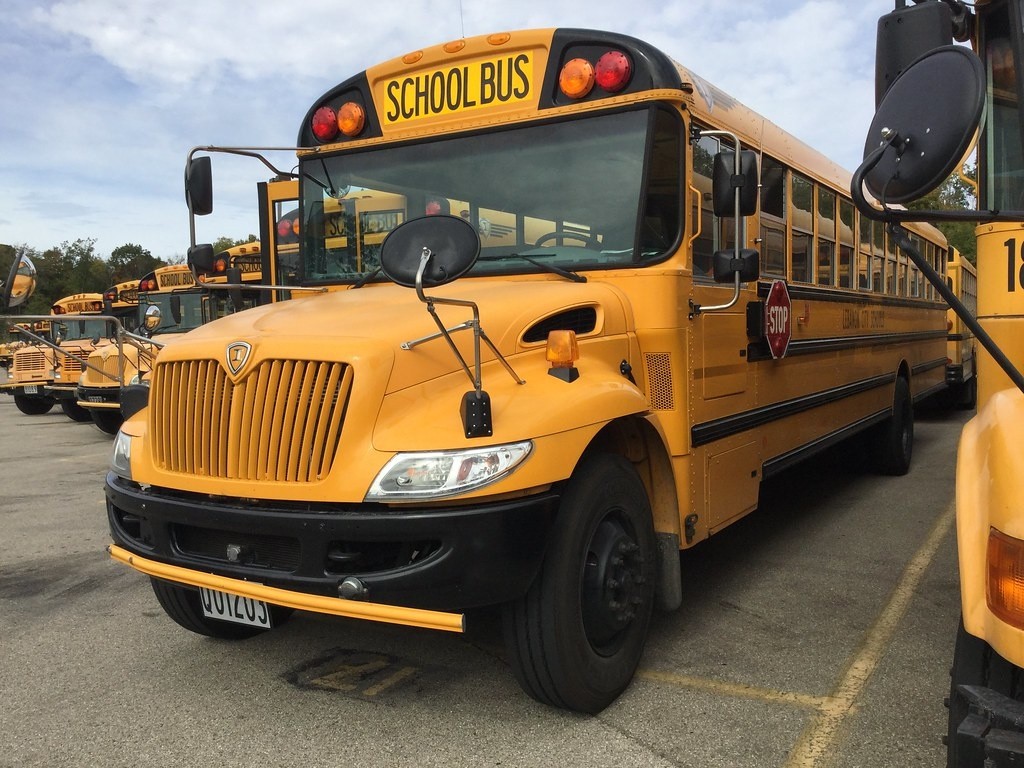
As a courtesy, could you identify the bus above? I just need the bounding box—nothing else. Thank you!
[946,243,977,411]
[102,25,949,716]
[848,1,1024,768]
[0,186,605,437]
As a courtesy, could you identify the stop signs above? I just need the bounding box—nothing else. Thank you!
[763,278,793,361]
